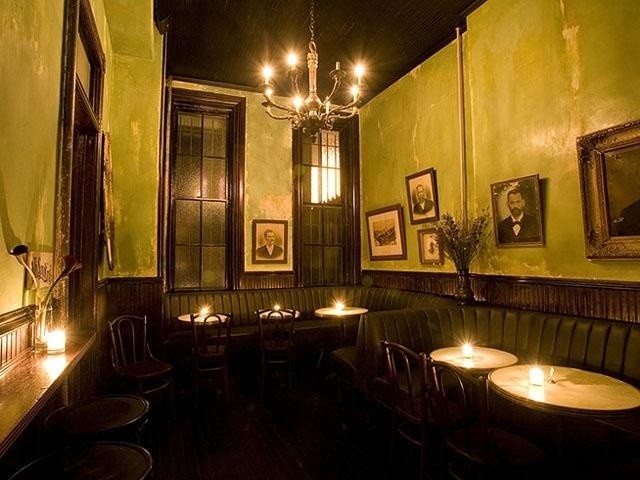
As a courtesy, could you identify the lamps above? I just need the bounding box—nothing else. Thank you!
[253,0,371,146]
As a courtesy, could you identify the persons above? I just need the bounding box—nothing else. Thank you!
[258,230,283,259]
[499,189,541,242]
[413,185,433,214]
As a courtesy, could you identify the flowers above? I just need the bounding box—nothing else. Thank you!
[429,207,494,268]
[9,243,82,341]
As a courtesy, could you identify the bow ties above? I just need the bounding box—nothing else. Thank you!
[511,220,521,228]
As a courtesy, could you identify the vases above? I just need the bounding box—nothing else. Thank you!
[454,268,474,306]
[31,290,54,351]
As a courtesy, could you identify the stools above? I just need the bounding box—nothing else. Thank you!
[45,388,152,443]
[10,440,153,479]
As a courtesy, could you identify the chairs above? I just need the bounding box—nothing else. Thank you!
[379,339,551,476]
[104,302,373,432]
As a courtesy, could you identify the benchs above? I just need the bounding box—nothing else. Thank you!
[161,284,457,382]
[365,299,640,480]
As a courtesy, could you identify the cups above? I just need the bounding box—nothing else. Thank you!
[461,343,473,358]
[529,366,545,387]
[45,332,66,355]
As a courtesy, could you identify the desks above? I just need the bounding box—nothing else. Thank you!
[1,324,97,461]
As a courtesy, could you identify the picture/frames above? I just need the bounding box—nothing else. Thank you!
[364,202,407,262]
[415,228,445,266]
[251,218,289,264]
[575,121,640,264]
[489,174,546,250]
[403,167,442,225]
